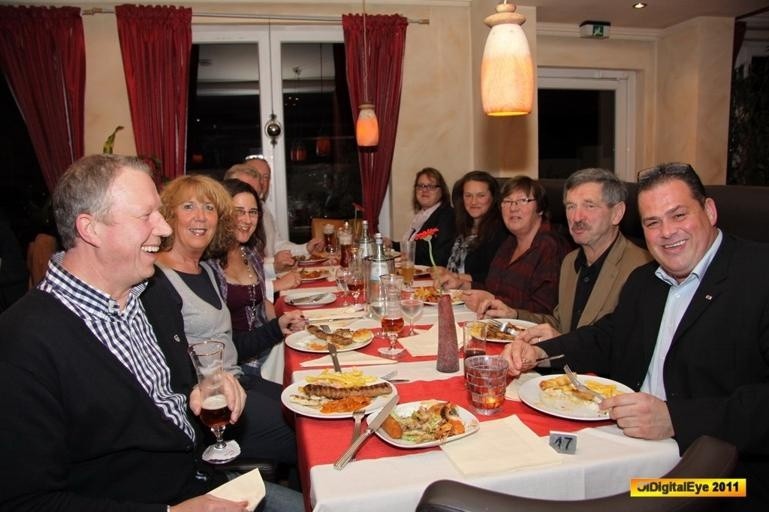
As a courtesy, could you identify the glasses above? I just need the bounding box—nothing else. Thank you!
[416,183,440,191]
[500,198,535,208]
[234,209,260,217]
[637,163,709,197]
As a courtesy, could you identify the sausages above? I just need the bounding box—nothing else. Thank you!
[382,416,402,439]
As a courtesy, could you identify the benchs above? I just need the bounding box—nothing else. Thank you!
[492,176,769,251]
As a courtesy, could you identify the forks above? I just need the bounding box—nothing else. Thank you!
[349,408,366,462]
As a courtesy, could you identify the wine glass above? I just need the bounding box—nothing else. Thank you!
[187,340,240,466]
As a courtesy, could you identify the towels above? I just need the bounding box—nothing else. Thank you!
[396,318,471,357]
[279,287,342,295]
[439,414,561,475]
[299,349,397,367]
[301,306,369,319]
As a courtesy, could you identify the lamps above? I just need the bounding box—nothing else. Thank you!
[355,0,379,147]
[480,0,536,117]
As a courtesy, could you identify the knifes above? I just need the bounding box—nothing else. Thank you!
[334,393,400,471]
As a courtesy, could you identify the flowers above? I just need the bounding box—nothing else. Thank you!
[413,228,444,288]
[352,203,365,234]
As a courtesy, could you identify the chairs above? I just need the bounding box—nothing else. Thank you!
[310,216,346,241]
[417,434,738,512]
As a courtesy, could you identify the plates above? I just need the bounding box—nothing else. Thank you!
[279,220,636,449]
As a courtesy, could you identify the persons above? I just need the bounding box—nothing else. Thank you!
[0,154,768,510]
